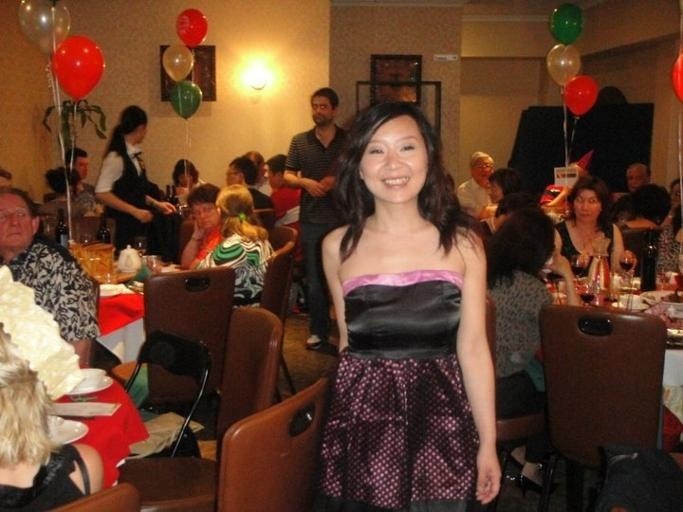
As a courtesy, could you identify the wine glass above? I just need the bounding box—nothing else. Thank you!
[542,250,638,307]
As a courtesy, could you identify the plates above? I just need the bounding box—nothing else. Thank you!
[99,284,123,298]
[544,271,683,339]
[48,418,90,445]
[65,376,113,397]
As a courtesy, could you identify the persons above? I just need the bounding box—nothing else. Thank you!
[0,186,99,375]
[0,322,105,511]
[479,207,560,497]
[455,146,681,277]
[313,100,503,512]
[38,86,350,351]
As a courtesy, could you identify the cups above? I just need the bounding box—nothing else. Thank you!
[143,255,163,278]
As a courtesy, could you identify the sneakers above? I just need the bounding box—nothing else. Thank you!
[306,335,321,350]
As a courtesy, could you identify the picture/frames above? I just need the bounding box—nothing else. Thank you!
[355,81,440,131]
[370,52,421,106]
[159,45,216,102]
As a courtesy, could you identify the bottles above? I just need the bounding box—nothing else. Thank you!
[96,213,112,244]
[164,184,182,220]
[54,207,70,249]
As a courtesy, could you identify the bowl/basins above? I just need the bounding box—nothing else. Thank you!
[48,415,65,432]
[78,367,108,389]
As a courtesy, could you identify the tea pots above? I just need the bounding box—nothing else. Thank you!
[117,244,142,273]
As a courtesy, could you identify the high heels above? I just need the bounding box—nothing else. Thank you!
[519,475,558,497]
[502,457,521,484]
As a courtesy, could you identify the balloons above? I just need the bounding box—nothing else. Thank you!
[673,54,683,100]
[161,7,208,121]
[546,3,599,119]
[18,1,106,100]
